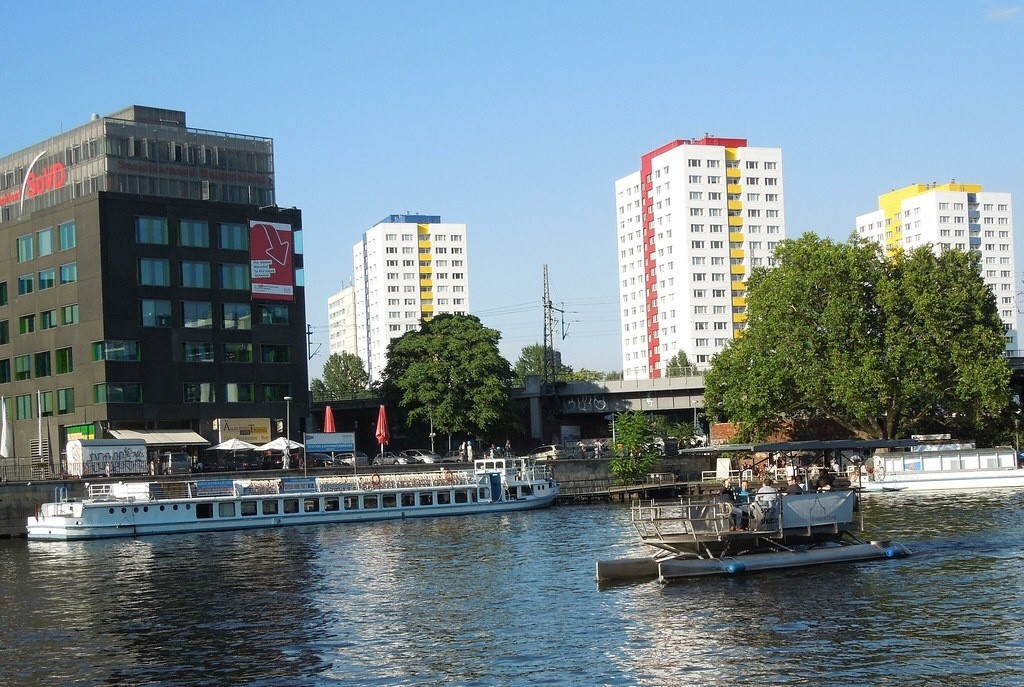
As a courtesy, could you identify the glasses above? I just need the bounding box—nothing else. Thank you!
[788,480,793,482]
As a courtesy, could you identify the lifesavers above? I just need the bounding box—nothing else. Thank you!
[444,470,453,482]
[371,474,380,485]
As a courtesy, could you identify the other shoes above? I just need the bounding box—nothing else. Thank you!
[736,528,743,531]
[730,526,734,530]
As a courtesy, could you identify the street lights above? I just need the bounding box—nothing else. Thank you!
[1013,419,1020,453]
[692,400,698,425]
[284,396,293,452]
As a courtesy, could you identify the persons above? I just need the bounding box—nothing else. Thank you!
[506,440,511,457]
[720,480,743,531]
[785,475,802,495]
[737,482,756,513]
[795,475,806,494]
[829,460,839,472]
[816,469,835,486]
[461,441,466,461]
[816,477,832,494]
[750,479,777,512]
[576,440,586,459]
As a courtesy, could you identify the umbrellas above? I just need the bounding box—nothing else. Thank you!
[253,437,305,451]
[204,438,259,463]
[375,405,390,464]
[324,406,336,458]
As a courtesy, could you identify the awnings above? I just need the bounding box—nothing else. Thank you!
[109,430,211,447]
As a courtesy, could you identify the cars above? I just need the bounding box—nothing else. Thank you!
[444,451,467,463]
[373,450,443,466]
[216,451,370,471]
[1018,452,1024,468]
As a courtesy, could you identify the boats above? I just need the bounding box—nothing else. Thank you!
[26,478,565,541]
[594,440,921,585]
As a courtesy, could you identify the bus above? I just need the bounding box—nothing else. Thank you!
[158,451,189,475]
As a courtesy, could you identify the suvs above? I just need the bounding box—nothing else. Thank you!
[527,444,566,461]
[565,446,596,458]
